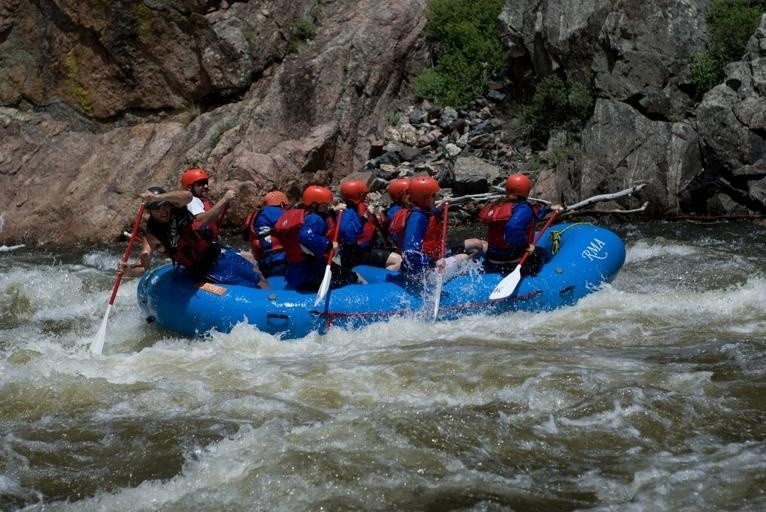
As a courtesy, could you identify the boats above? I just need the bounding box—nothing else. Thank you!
[137,219,626,341]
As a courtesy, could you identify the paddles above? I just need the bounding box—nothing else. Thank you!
[489,209,559,300]
[313,211,343,305]
[432,200,448,321]
[90,203,143,354]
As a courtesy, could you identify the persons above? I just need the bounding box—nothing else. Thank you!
[481,172,565,279]
[116,166,489,294]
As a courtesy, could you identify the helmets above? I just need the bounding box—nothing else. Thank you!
[505,173,533,200]
[141,187,169,209]
[410,176,440,208]
[301,184,335,207]
[179,167,210,189]
[340,180,371,206]
[262,191,291,207]
[388,178,410,202]
